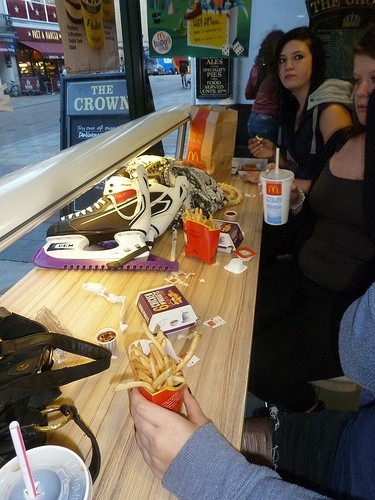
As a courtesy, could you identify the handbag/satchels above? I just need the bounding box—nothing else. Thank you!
[123,149,229,233]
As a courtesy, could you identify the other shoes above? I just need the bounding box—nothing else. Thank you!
[254,400,326,419]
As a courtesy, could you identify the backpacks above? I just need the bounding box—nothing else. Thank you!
[1,306,113,483]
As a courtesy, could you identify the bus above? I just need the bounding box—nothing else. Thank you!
[154,57,191,75]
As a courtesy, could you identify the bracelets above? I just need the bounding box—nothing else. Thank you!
[291,187,306,210]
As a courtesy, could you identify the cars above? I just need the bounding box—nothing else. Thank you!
[146,64,166,76]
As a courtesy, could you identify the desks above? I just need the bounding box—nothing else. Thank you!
[0,157,264,500]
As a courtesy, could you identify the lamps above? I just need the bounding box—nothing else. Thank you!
[1,103,193,254]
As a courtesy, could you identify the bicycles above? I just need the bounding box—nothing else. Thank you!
[1,80,22,97]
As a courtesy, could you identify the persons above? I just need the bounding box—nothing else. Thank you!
[243,25,375,413]
[127,90,375,500]
[247,27,357,269]
[120,57,125,72]
[245,29,285,163]
[180,60,189,88]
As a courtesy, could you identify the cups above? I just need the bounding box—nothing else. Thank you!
[95,328,118,353]
[225,211,237,222]
[0,445,93,500]
[260,169,294,225]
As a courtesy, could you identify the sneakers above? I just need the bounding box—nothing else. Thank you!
[34,163,180,272]
[94,173,189,261]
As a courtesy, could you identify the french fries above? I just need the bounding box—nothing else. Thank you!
[114,321,200,392]
[180,207,215,230]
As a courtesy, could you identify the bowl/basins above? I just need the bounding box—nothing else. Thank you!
[170,320,178,326]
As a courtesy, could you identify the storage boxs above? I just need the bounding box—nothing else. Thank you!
[136,286,199,336]
[128,338,187,415]
[212,218,244,254]
[182,210,220,264]
